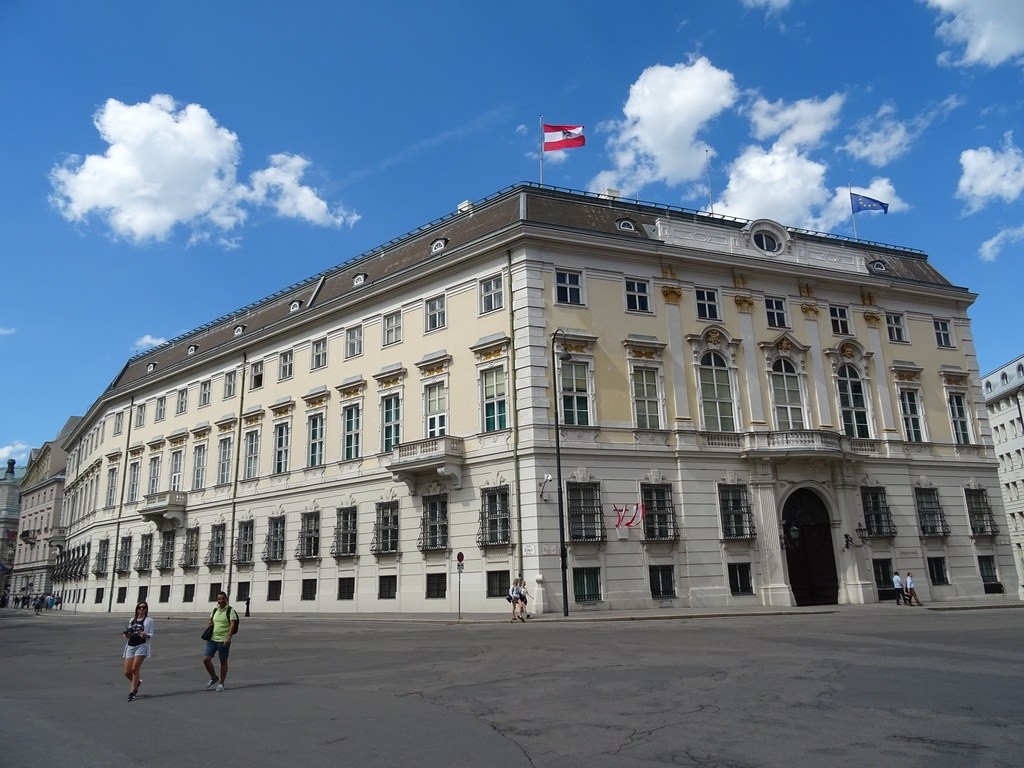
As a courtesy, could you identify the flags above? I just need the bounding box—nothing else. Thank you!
[849,192,890,216]
[540,122,586,153]
[6,529,15,541]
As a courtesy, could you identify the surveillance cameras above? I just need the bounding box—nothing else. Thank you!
[548,476,552,482]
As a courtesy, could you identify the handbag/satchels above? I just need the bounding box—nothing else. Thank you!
[124,622,144,638]
[226,606,239,634]
[506,595,512,603]
[200,608,217,640]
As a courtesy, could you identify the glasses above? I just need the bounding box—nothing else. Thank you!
[137,605,147,609]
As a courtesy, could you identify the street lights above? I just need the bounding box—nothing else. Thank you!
[552,326,573,621]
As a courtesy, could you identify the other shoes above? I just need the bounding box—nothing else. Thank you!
[206,677,219,687]
[137,679,142,688]
[126,692,136,701]
[517,615,522,619]
[512,617,517,620]
[215,683,224,691]
[527,615,530,618]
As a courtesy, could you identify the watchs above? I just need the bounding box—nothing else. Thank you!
[227,636,231,639]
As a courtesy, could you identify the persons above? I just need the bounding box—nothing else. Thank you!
[0,592,62,615]
[122,601,154,702]
[202,590,237,690]
[906,572,924,607]
[509,578,534,622]
[893,571,908,605]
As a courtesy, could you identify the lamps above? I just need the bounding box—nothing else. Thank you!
[844,521,867,549]
[779,520,800,551]
